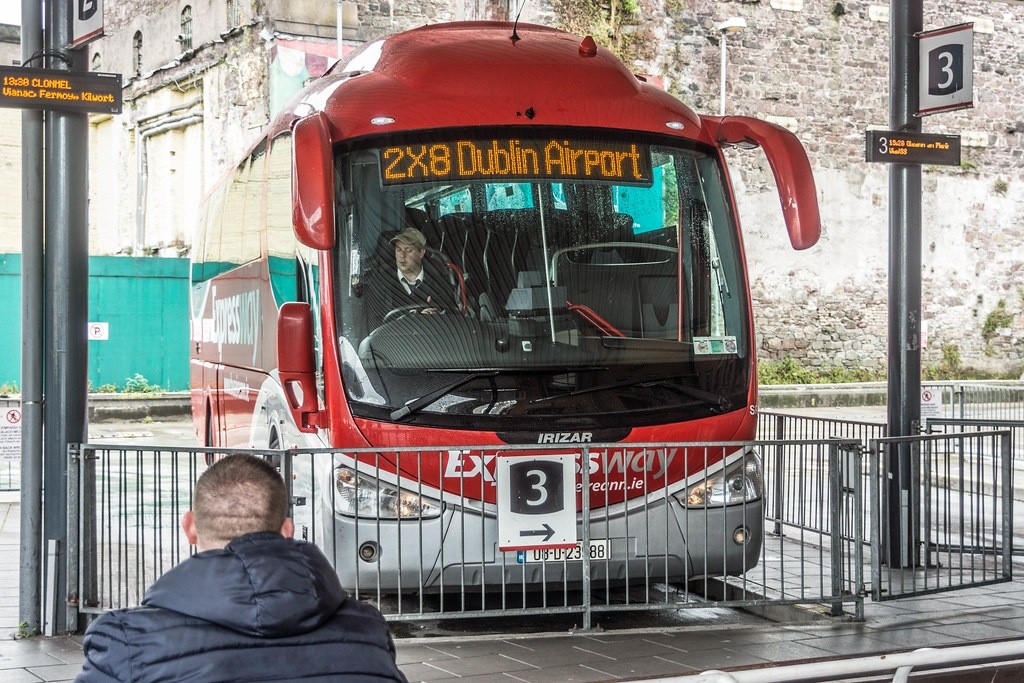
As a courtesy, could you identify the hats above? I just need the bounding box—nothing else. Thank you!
[389,226,426,247]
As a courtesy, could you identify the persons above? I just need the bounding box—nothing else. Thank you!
[364,228,460,335]
[72,453,410,683]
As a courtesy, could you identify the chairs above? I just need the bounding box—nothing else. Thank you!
[354,230,450,347]
[405,207,636,320]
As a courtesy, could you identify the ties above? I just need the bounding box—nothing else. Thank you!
[401,277,421,293]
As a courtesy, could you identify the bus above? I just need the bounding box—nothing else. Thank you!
[187,19,819,609]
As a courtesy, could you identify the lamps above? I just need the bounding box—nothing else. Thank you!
[832,3,845,16]
[175,32,186,43]
